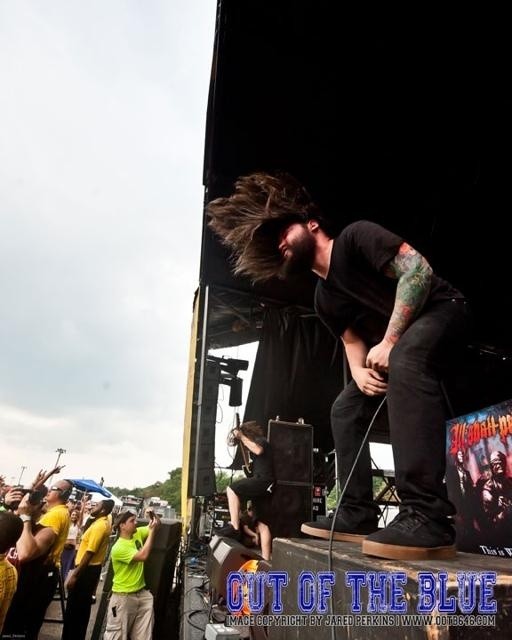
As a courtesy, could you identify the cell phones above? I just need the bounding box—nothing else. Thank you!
[148,510,154,522]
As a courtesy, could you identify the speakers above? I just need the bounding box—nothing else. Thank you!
[258,419,314,553]
[205,534,272,639]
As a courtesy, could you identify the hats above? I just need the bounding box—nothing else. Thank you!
[112,510,134,526]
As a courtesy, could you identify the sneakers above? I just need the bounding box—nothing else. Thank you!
[300,506,378,543]
[362,511,457,561]
[214,524,241,537]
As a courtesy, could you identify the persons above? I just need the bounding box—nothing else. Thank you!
[0,466,162,640]
[207,174,472,560]
[480,452,512,548]
[445,450,474,503]
[241,507,258,547]
[216,423,275,563]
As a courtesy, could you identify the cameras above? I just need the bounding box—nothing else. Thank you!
[9,488,41,510]
